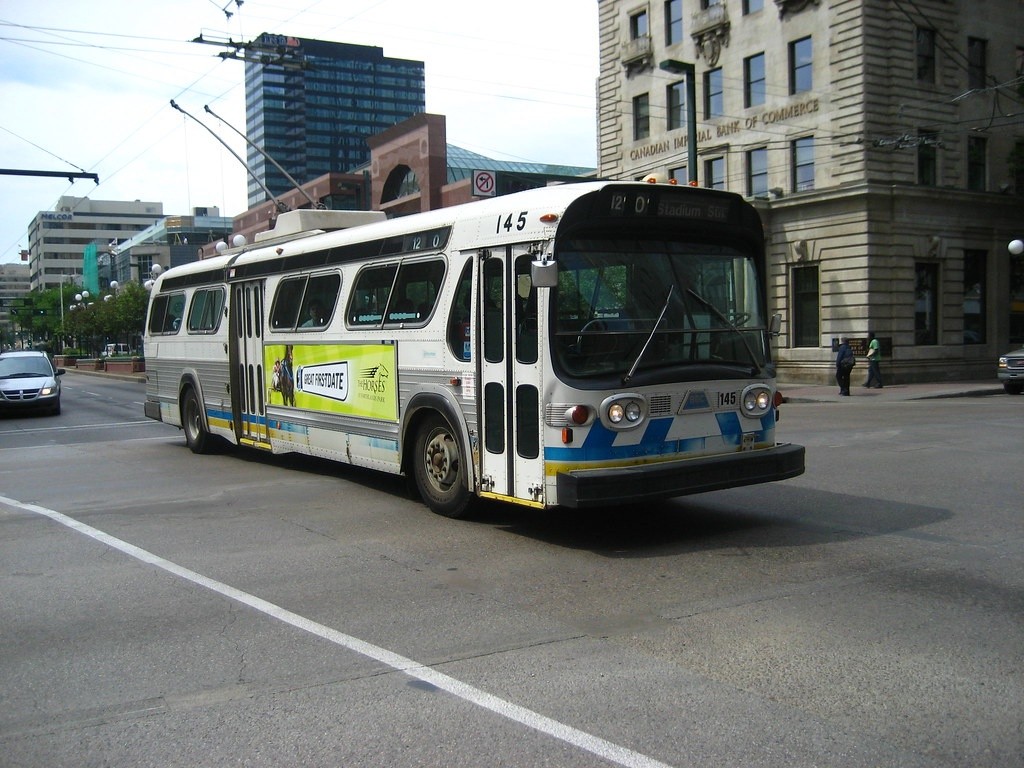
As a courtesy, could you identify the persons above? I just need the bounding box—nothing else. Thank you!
[835,337,855,396]
[300,297,326,327]
[272,361,282,390]
[861,331,883,388]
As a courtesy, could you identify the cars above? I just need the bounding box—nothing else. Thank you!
[996,345,1024,394]
[0,350,66,417]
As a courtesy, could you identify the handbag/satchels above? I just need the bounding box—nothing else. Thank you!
[840,356,855,368]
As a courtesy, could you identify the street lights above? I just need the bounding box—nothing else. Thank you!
[658,59,698,188]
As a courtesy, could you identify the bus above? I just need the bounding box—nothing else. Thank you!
[142,175,808,522]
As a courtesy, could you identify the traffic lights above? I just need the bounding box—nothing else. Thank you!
[33,309,46,315]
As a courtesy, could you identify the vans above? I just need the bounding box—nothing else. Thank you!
[104,344,132,357]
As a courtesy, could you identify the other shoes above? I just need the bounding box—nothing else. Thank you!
[839,389,848,395]
[861,384,870,388]
[875,385,882,388]
